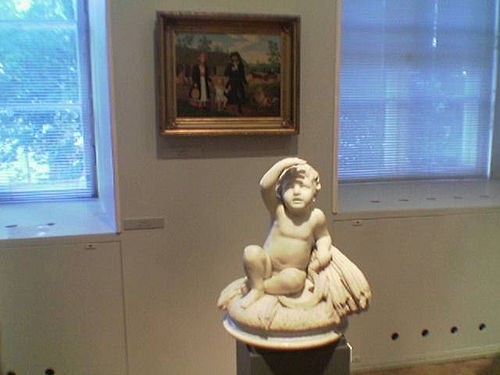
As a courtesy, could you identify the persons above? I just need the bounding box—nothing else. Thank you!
[236,157,332,309]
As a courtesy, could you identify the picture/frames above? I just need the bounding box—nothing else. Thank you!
[155,10,300,138]
